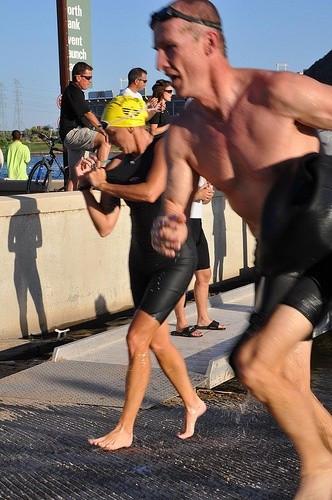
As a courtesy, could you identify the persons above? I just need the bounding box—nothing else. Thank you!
[0,148,5,172]
[73,95,207,451]
[59,60,175,192]
[151,0,332,500]
[6,129,31,180]
[175,176,224,337]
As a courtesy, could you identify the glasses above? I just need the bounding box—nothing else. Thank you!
[76,75,92,80]
[150,5,222,30]
[100,120,109,130]
[136,78,147,83]
[164,90,173,94]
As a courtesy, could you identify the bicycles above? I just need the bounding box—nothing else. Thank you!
[25,131,69,194]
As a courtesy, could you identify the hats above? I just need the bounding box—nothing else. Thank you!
[101,95,148,127]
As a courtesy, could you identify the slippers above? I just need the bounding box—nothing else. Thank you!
[171,326,203,337]
[194,320,225,330]
